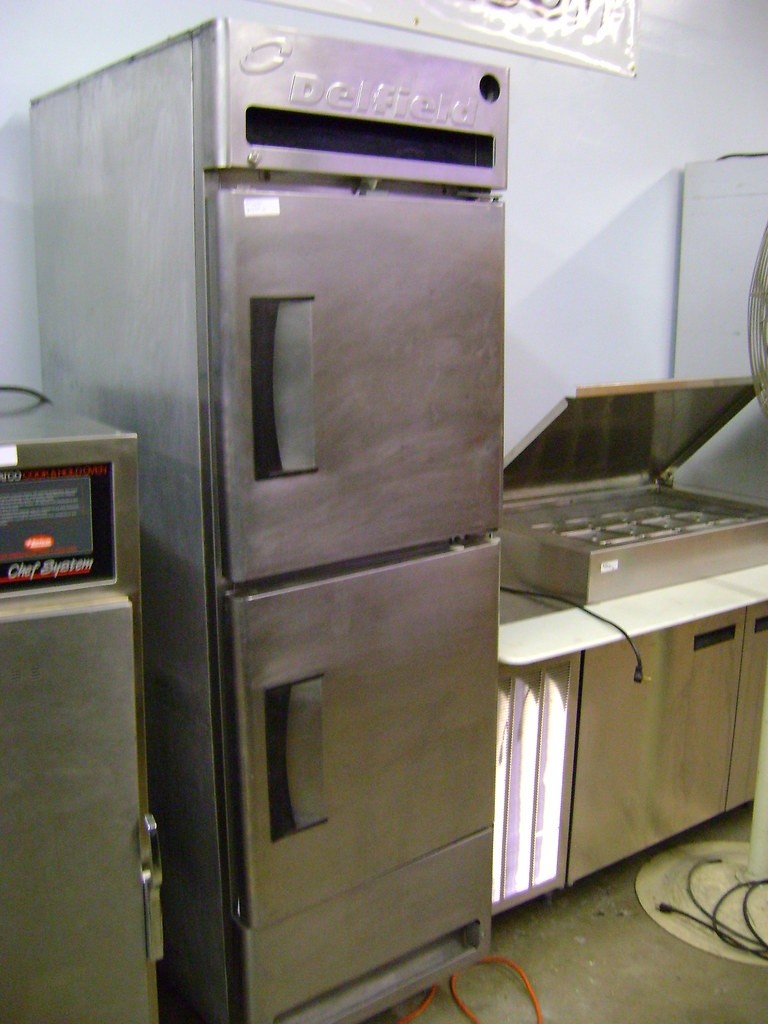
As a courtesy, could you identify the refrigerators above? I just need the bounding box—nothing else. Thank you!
[29,21,512,1024]
